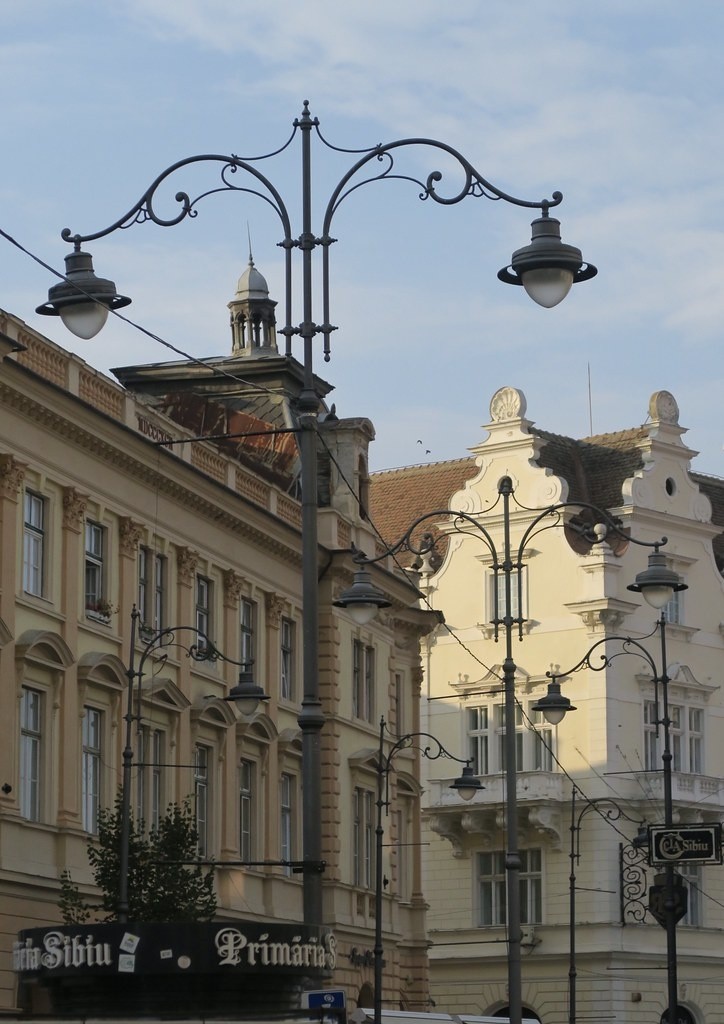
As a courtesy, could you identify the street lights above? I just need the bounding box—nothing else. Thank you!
[369,714,489,1024]
[114,604,272,923]
[331,471,689,1024]
[528,609,681,1024]
[565,781,649,1024]
[30,100,601,989]
